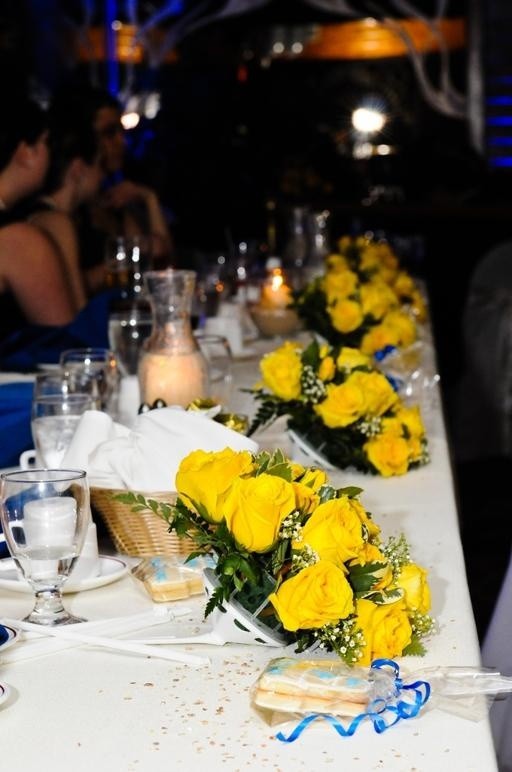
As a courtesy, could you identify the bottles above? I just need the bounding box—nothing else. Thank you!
[137,267,212,410]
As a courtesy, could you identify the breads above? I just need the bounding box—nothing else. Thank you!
[254,656,369,719]
[146,570,206,601]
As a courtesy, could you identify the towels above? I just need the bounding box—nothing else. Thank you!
[53,404,259,495]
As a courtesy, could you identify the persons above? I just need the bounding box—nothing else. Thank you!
[0,76,87,469]
[1,106,134,372]
[56,84,177,274]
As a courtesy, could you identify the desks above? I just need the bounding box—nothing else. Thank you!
[0,279,501,772]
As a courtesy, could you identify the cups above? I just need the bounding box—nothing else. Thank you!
[110,207,333,377]
[20,348,114,474]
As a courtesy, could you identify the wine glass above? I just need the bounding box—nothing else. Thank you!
[0,469,93,627]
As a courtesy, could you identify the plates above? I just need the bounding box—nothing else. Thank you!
[0,557,128,594]
